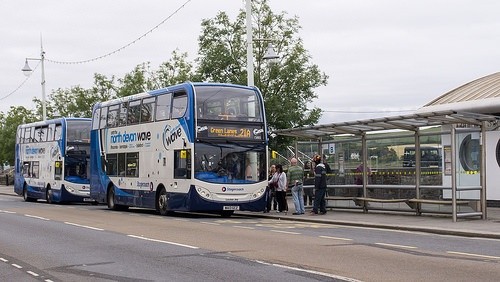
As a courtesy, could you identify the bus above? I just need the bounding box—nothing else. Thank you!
[89,79,277,218]
[14,116,99,206]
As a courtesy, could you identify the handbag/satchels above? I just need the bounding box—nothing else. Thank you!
[273,182,278,188]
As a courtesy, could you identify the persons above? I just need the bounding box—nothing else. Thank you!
[322,154,333,185]
[245,158,252,181]
[287,158,305,214]
[311,154,328,215]
[268,164,288,213]
[302,161,314,206]
[352,161,371,206]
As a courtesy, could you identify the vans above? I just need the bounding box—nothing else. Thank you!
[403,146,447,167]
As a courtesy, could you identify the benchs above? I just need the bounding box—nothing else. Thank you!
[312,197,470,216]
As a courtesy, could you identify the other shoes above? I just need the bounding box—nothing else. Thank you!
[264,208,267,213]
[300,213,304,214]
[320,211,327,215]
[310,211,318,214]
[292,213,300,215]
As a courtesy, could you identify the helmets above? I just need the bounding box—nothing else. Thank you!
[314,155,321,162]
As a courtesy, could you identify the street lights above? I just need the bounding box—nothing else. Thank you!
[20,49,47,121]
[246,37,281,181]
